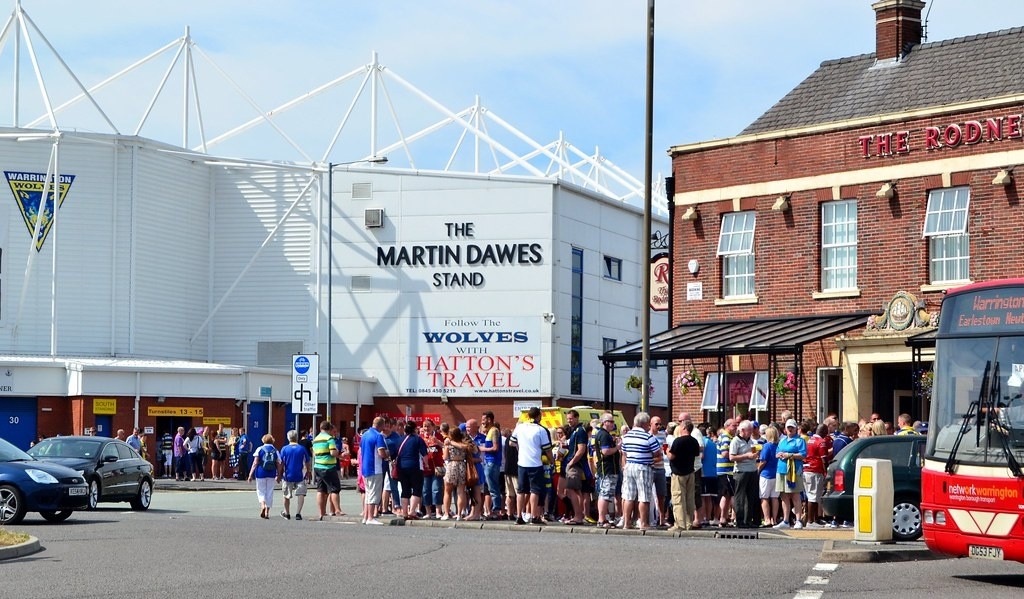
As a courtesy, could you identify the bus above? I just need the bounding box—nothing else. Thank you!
[919,278,1023,565]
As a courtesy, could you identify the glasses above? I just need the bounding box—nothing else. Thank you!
[678,420,685,422]
[873,418,882,420]
[89,431,94,433]
[606,420,615,424]
[655,423,662,426]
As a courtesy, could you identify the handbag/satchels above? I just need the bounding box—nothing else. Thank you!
[195,447,205,456]
[566,467,586,490]
[388,460,398,479]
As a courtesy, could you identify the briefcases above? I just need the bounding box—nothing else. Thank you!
[434,467,446,477]
[467,458,479,485]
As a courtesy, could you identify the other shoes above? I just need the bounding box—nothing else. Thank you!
[320,512,348,521]
[596,514,849,531]
[516,512,596,524]
[361,510,517,520]
[212,474,224,480]
[176,477,204,481]
[260,509,269,519]
[162,474,171,478]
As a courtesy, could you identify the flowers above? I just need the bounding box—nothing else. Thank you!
[677,371,700,397]
[641,384,654,398]
[774,370,800,394]
[914,370,932,402]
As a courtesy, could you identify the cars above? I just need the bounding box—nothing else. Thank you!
[821,434,929,541]
[24,436,155,511]
[0,438,89,525]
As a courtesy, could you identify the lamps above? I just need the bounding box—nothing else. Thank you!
[651,231,667,248]
[681,166,1014,220]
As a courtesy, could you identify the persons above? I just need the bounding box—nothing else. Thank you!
[89,427,96,436]
[300,427,350,479]
[351,408,920,529]
[38,433,48,441]
[247,434,282,520]
[311,421,346,520]
[279,430,311,520]
[161,423,252,483]
[30,441,37,447]
[115,429,124,440]
[56,432,63,436]
[126,427,147,460]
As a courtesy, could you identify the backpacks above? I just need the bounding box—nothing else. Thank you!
[259,446,277,471]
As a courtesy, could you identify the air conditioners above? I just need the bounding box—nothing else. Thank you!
[365,209,382,227]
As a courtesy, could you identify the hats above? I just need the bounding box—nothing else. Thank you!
[914,422,928,432]
[785,418,798,427]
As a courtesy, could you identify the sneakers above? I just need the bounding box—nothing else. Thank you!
[280,511,290,520]
[296,514,302,520]
[362,518,384,525]
